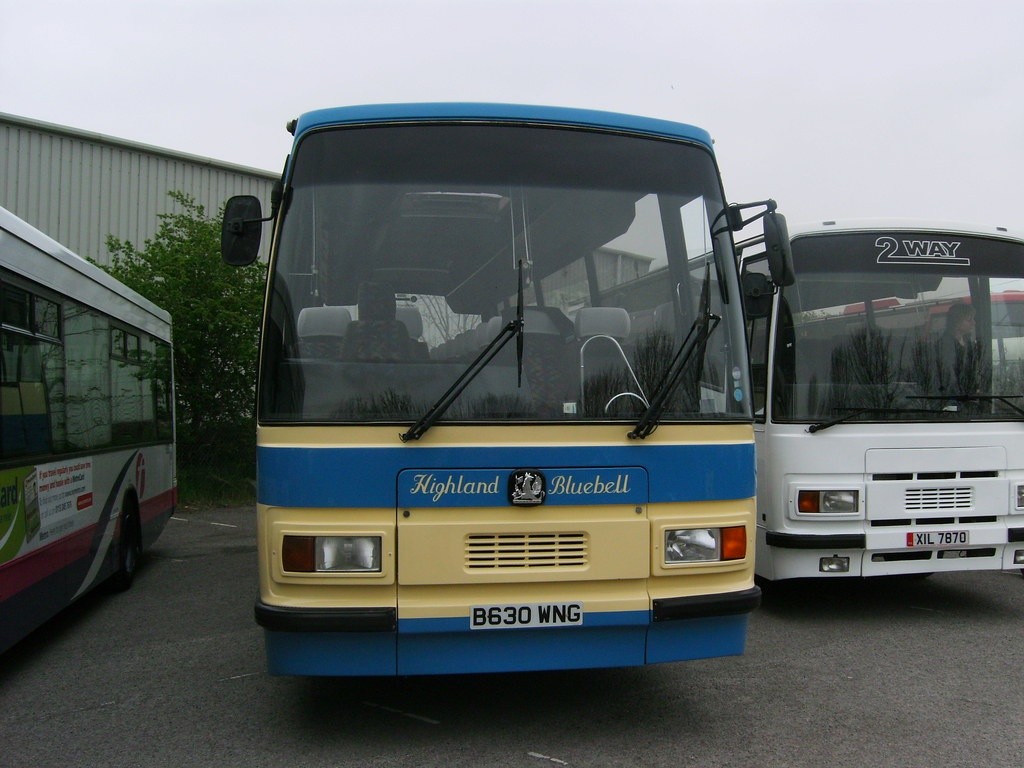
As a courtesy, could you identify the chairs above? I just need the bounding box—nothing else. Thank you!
[297,306,632,413]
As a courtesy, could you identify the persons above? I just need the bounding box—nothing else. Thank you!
[931,304,983,411]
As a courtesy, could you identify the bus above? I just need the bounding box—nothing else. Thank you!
[0,205,181,656]
[567,219,1023,596]
[218,100,796,684]
